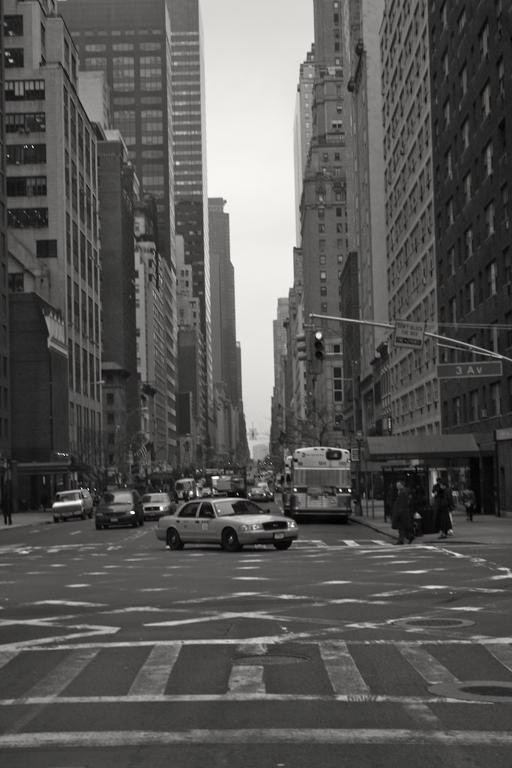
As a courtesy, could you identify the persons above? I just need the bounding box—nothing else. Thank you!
[1,493,16,525]
[387,470,479,545]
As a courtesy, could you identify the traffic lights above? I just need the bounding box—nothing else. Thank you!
[295,323,324,360]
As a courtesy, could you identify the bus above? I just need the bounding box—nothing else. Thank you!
[282,447,351,525]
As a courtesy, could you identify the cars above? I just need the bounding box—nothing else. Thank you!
[52,475,298,551]
